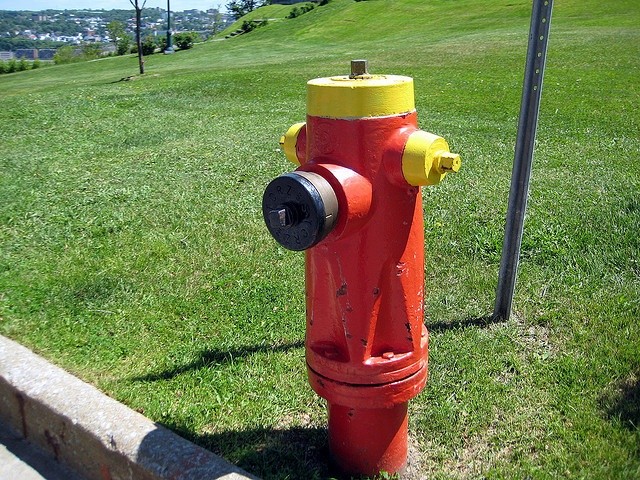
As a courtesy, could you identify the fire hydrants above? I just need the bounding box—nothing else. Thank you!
[263,59,460,473]
